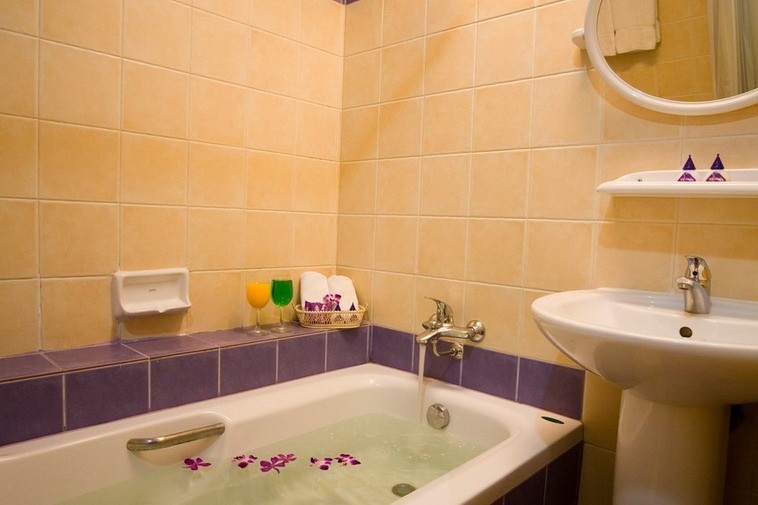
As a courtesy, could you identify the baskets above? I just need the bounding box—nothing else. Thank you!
[290,300,369,329]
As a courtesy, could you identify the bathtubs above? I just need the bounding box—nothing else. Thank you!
[0,358,586,505]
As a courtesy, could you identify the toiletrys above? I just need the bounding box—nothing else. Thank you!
[675,154,702,183]
[702,153,731,182]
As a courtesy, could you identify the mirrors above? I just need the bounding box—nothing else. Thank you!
[572,2,758,118]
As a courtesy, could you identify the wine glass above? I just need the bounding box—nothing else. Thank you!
[268,269,294,333]
[245,276,269,337]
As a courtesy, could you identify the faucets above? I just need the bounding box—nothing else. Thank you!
[414,295,460,347]
[674,255,714,315]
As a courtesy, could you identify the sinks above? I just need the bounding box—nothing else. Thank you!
[531,285,758,406]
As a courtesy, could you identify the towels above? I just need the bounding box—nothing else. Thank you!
[298,271,331,323]
[596,1,617,58]
[328,273,360,325]
[613,0,662,54]
